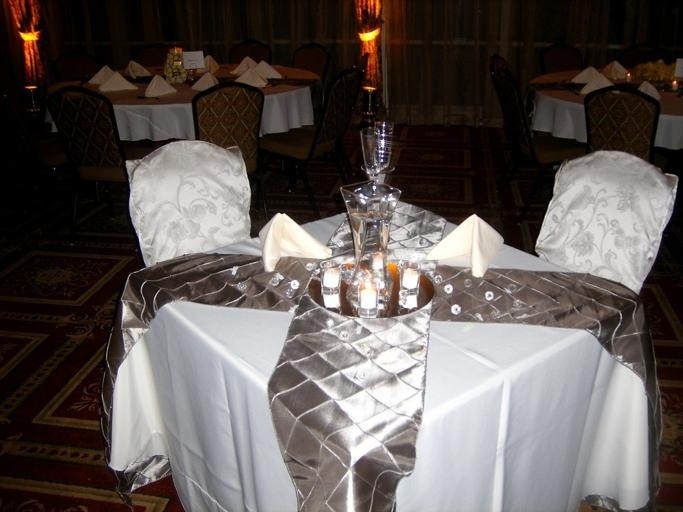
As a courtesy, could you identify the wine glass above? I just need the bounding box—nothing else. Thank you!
[188,63,197,82]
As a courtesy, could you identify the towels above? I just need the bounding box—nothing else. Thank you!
[256,209,334,274]
[428,207,506,282]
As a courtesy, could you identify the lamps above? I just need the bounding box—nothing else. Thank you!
[354,10,383,45]
[19,17,39,43]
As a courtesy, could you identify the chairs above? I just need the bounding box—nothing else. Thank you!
[122,137,253,267]
[533,149,681,298]
[483,48,683,233]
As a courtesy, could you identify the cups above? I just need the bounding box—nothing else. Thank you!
[398,262,420,296]
[357,281,377,318]
[319,260,341,296]
